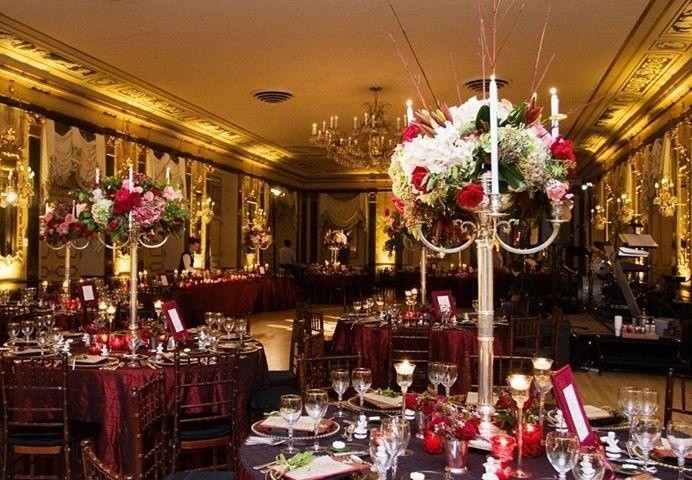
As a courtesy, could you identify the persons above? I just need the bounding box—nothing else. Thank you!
[180,237,199,275]
[277,239,295,262]
[492,244,505,267]
[509,261,532,315]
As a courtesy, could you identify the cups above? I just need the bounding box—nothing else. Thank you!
[406,393,545,480]
[614,316,622,336]
[567,445,606,479]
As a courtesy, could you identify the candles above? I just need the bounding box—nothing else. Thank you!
[152,299,164,316]
[95,168,100,183]
[506,373,534,409]
[166,167,171,183]
[393,358,417,394]
[490,76,501,195]
[71,200,75,216]
[549,90,559,140]
[531,357,555,395]
[106,303,117,323]
[407,99,412,126]
[46,202,49,212]
[97,301,107,315]
[129,165,134,227]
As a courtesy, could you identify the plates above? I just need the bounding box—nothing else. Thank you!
[3,324,260,366]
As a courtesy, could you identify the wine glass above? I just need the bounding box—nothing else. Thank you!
[369,416,411,479]
[384,299,478,330]
[629,413,661,480]
[8,310,248,356]
[354,292,384,320]
[666,421,692,480]
[279,393,303,454]
[303,388,330,453]
[427,360,460,398]
[545,430,580,480]
[329,368,350,417]
[616,384,660,420]
[0,287,37,306]
[351,368,373,418]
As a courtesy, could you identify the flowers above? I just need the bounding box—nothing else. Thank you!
[392,96,579,247]
[90,168,185,244]
[40,173,95,247]
[323,228,349,250]
[241,222,275,254]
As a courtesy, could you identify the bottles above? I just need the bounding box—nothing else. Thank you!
[644,319,655,332]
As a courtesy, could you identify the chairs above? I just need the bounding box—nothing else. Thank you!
[5,351,74,477]
[79,436,126,478]
[156,340,241,476]
[130,371,239,479]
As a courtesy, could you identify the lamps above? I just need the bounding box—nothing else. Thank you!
[588,205,612,230]
[654,176,689,217]
[617,195,644,224]
[2,164,33,208]
[257,208,265,222]
[194,197,215,223]
[311,88,407,172]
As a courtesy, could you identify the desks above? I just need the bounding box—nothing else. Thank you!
[237,387,692,480]
[5,328,268,473]
[339,306,504,385]
[3,266,297,478]
[291,265,691,378]
